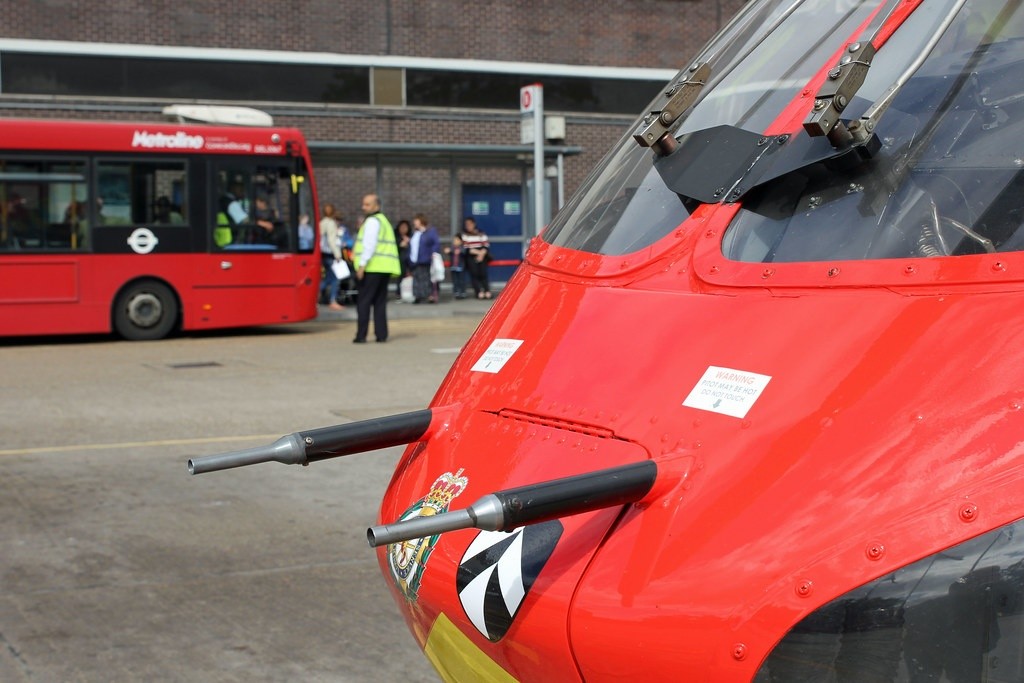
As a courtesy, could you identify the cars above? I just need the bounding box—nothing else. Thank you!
[184,0,1024,683]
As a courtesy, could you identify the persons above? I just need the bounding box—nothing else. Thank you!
[445,217,492,299]
[394,214,440,302]
[154,195,182,226]
[320,204,364,309]
[350,194,401,343]
[257,195,313,248]
[64,198,105,223]
[222,182,273,230]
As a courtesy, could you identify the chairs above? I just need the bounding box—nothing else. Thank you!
[13,218,81,250]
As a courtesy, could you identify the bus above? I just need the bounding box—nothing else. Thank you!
[1,98,323,342]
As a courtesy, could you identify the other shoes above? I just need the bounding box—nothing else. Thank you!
[330,303,342,309]
[455,292,460,298]
[461,292,465,298]
[478,292,485,299]
[427,296,435,302]
[376,339,385,342]
[353,338,366,343]
[485,291,492,298]
[413,298,420,303]
